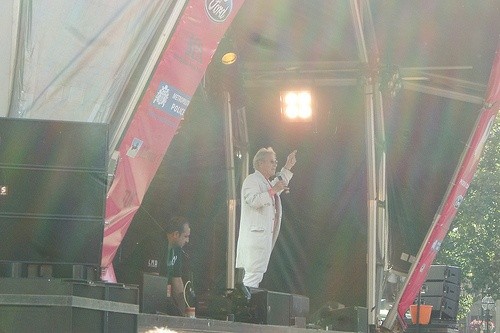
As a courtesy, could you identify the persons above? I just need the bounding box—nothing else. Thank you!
[116,215,191,317]
[235,147,298,288]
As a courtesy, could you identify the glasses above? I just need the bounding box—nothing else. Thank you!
[261,159,279,164]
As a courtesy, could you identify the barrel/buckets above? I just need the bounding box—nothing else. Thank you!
[410,305,433,325]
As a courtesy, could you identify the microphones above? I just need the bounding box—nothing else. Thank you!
[276,172,289,194]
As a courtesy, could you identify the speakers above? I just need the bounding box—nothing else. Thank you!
[0,116,109,266]
[251,291,292,326]
[413,265,461,320]
[290,294,310,329]
[321,306,368,332]
[139,273,169,314]
